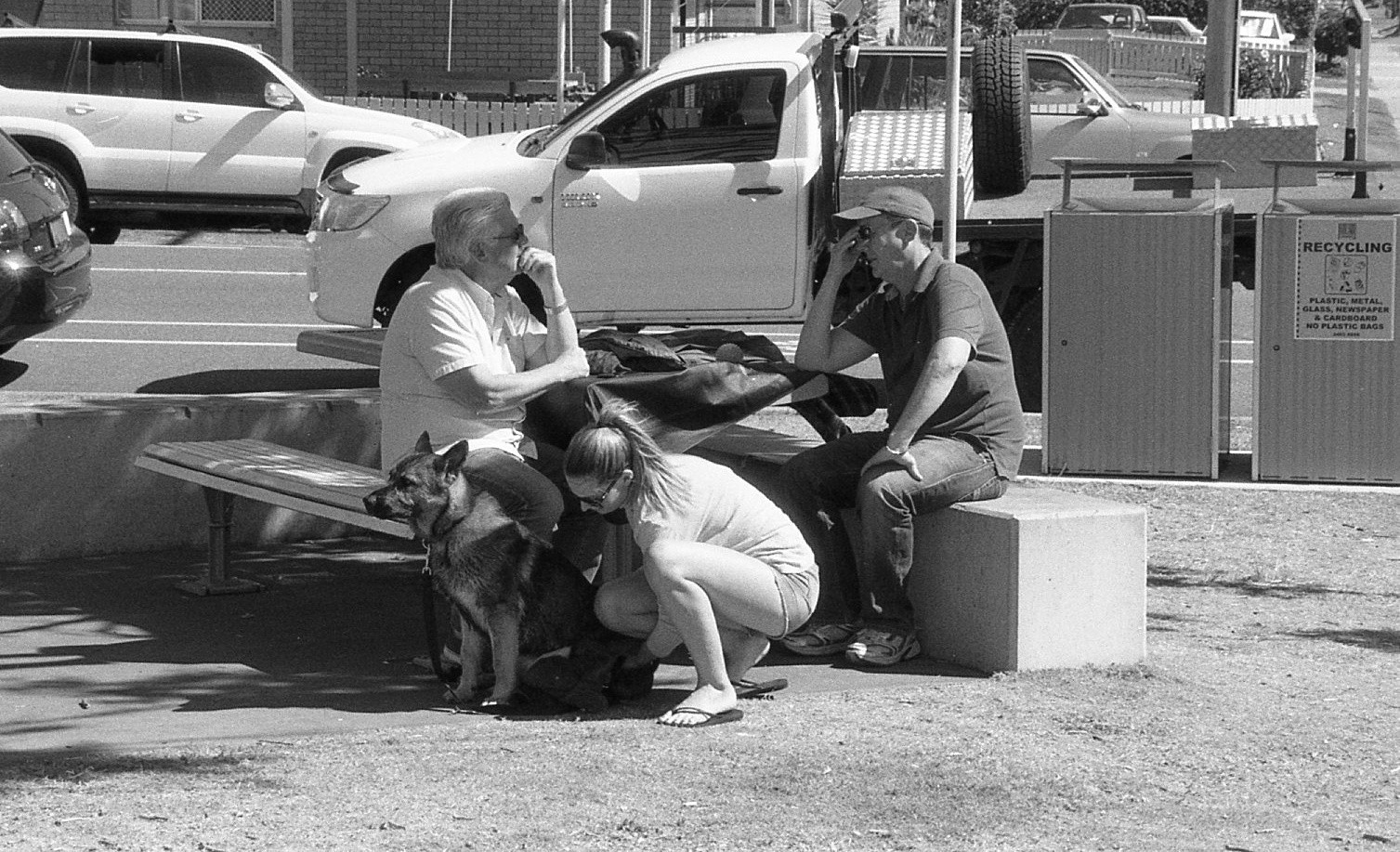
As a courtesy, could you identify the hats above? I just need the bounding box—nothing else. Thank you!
[833,185,936,232]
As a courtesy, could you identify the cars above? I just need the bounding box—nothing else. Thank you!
[1190,10,1295,50]
[1,130,95,355]
[1052,3,1204,38]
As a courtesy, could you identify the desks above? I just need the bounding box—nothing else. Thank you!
[296,328,828,581]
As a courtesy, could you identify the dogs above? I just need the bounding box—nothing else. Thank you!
[362,429,655,717]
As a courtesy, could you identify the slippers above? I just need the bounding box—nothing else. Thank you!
[731,679,787,699]
[665,708,743,728]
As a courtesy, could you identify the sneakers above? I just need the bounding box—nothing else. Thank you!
[784,622,863,657]
[845,630,919,667]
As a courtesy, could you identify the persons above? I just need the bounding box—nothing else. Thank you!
[781,187,1025,665]
[378,189,612,586]
[561,398,820,728]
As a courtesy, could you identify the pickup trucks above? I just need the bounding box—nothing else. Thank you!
[297,26,1400,413]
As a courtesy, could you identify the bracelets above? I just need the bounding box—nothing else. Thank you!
[886,444,909,455]
[543,300,568,315]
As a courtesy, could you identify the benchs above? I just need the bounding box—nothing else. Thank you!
[133,438,559,682]
[694,422,1149,677]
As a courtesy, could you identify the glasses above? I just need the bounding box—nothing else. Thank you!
[570,478,619,509]
[493,224,524,244]
[858,219,895,238]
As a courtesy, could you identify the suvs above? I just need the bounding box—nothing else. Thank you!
[0,12,468,247]
[832,42,1233,176]
